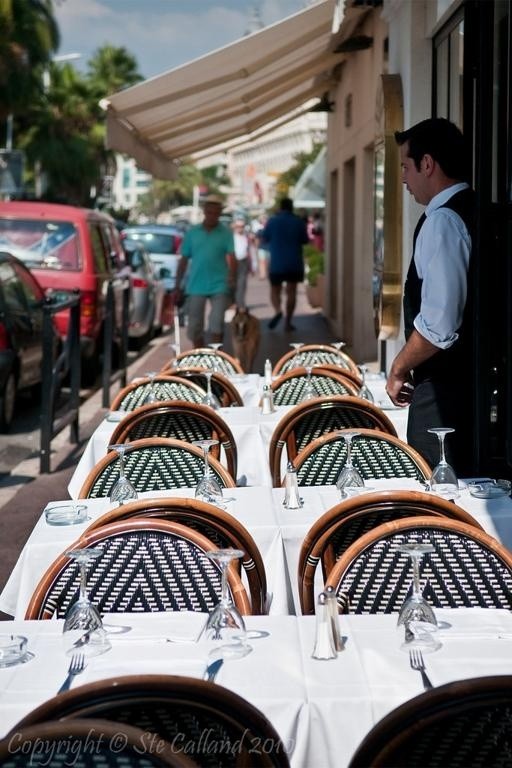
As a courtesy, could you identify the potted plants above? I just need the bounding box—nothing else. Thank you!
[301,244,326,311]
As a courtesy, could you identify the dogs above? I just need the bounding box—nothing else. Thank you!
[230,306,262,374]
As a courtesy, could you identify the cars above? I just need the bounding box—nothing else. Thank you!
[121,238,171,347]
[0,253,68,423]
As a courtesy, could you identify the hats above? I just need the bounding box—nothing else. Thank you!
[199,194,226,209]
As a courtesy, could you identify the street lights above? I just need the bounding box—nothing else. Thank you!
[42,49,83,95]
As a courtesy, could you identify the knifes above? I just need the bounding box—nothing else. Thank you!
[206,658,226,685]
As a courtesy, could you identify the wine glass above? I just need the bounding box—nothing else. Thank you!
[145,342,224,409]
[62,549,109,656]
[191,439,224,508]
[201,549,249,661]
[291,340,373,402]
[334,431,365,489]
[106,443,141,509]
[428,428,460,499]
[393,543,443,653]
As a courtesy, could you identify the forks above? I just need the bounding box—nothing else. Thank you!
[407,647,437,692]
[55,652,89,698]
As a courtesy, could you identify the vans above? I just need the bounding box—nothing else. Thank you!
[119,223,187,294]
[0,199,134,385]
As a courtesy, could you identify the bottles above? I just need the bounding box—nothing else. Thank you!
[311,585,340,661]
[282,459,305,509]
[262,383,275,415]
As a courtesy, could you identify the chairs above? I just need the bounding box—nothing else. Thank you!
[1,330,512,767]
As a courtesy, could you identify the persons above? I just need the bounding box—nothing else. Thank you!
[171,195,323,349]
[385,117,512,479]
